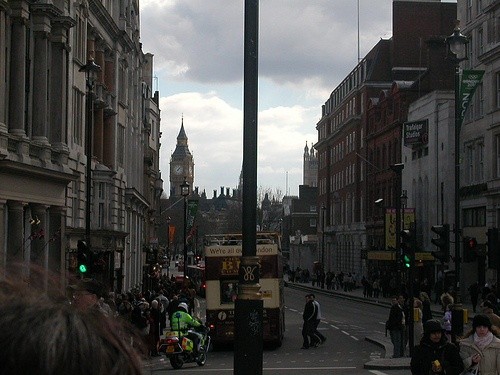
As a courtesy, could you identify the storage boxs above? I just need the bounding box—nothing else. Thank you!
[160,338,166,352]
[181,337,193,350]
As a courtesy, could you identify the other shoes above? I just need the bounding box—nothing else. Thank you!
[392,347,406,358]
[301,337,326,350]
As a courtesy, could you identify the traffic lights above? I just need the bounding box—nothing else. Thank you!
[431,223,450,262]
[462,237,478,262]
[437,269,459,291]
[399,228,416,269]
[76,239,92,279]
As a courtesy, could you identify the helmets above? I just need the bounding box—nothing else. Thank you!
[177,302,188,313]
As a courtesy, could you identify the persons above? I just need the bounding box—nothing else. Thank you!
[458,314,500,375]
[0,251,202,375]
[288,266,500,358]
[410,320,465,375]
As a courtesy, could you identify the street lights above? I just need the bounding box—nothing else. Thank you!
[179,175,191,279]
[79,55,103,292]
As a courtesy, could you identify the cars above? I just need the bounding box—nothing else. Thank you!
[174,253,206,299]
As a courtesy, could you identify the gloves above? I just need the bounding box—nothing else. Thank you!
[198,324,206,332]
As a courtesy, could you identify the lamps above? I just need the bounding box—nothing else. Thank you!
[24,215,36,224]
[25,217,41,224]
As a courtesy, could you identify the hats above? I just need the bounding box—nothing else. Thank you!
[473,314,491,327]
[68,277,102,296]
[423,319,446,341]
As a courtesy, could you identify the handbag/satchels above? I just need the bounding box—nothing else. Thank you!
[461,361,480,375]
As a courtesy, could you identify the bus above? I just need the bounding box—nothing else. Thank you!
[203,231,289,353]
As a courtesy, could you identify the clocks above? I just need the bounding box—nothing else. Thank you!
[174,164,184,175]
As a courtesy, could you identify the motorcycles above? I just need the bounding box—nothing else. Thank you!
[156,314,212,370]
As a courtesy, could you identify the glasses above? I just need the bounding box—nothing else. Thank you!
[72,293,93,299]
